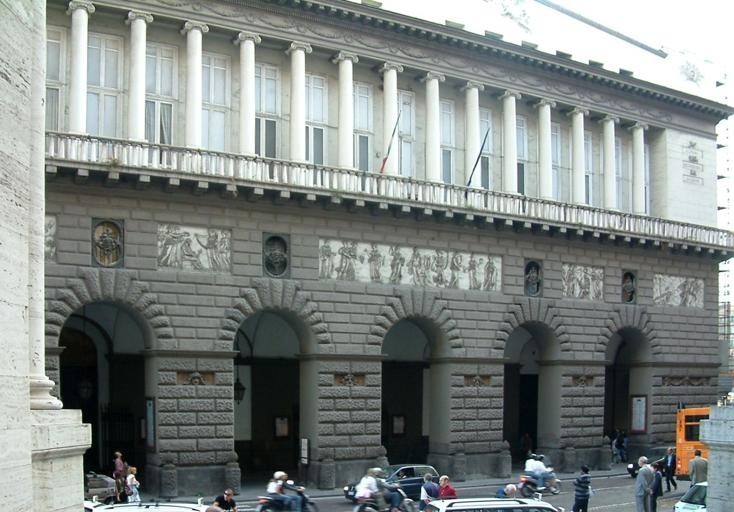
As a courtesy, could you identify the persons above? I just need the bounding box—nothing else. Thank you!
[653,273,704,308]
[571,464,590,511]
[354,468,391,512]
[536,454,556,492]
[266,471,290,511]
[373,467,401,512]
[318,237,499,292]
[561,263,603,298]
[397,469,407,477]
[438,474,456,499]
[688,449,707,489]
[604,427,631,464]
[204,504,224,511]
[280,471,305,511]
[494,483,516,497]
[112,449,126,503]
[649,462,662,511]
[519,431,531,469]
[418,472,439,512]
[212,487,238,511]
[154,223,228,272]
[633,455,655,512]
[523,454,546,490]
[663,446,677,492]
[125,465,141,503]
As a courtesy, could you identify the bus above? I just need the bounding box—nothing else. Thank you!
[675,407,709,475]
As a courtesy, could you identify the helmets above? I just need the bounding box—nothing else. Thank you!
[529,453,537,459]
[536,454,545,461]
[273,471,286,480]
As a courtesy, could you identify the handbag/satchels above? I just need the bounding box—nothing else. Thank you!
[124,480,133,496]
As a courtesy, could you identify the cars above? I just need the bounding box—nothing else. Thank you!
[627,446,676,477]
[344,464,440,502]
[84,471,227,512]
[672,482,708,512]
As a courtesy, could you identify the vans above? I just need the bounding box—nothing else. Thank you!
[420,493,565,512]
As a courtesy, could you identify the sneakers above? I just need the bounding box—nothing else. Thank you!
[536,486,546,491]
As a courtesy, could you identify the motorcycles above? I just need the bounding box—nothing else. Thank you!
[256,480,320,512]
[518,464,562,497]
[344,474,419,512]
